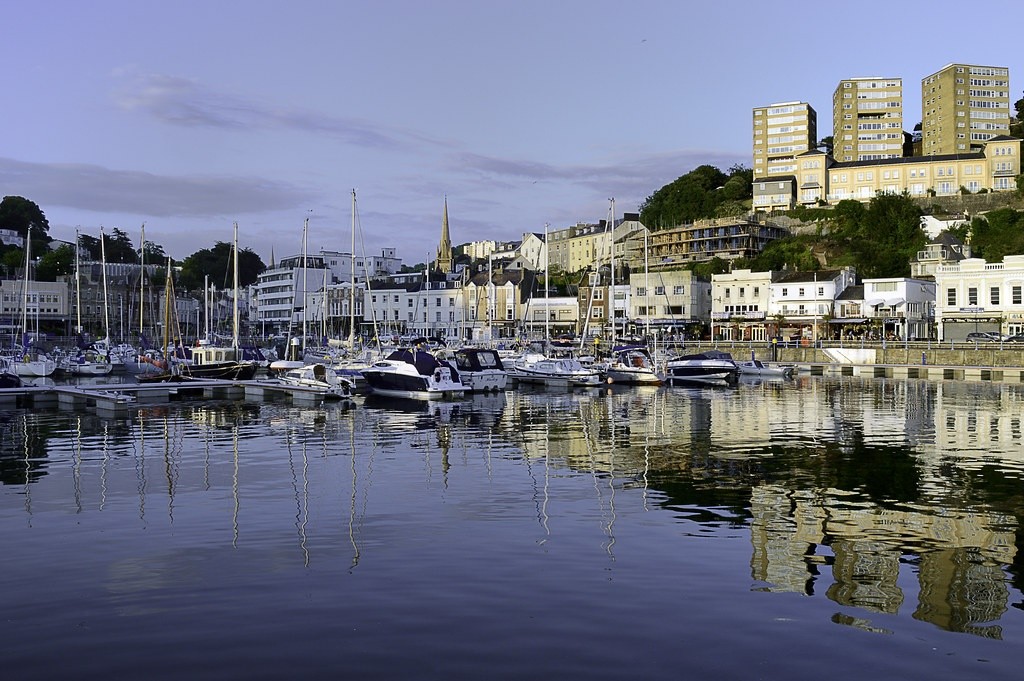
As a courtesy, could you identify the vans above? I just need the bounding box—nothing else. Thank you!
[272,331,295,341]
[28,329,47,338]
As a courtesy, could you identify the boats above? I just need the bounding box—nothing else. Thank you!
[739,359,795,375]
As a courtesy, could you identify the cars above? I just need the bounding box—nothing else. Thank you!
[621,334,644,341]
[298,332,314,342]
[378,333,398,341]
[987,332,1011,341]
[399,332,420,341]
[966,332,995,344]
[1004,333,1024,343]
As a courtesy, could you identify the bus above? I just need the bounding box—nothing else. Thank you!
[0,325,24,340]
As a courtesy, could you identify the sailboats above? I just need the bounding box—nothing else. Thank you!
[0,188,745,411]
